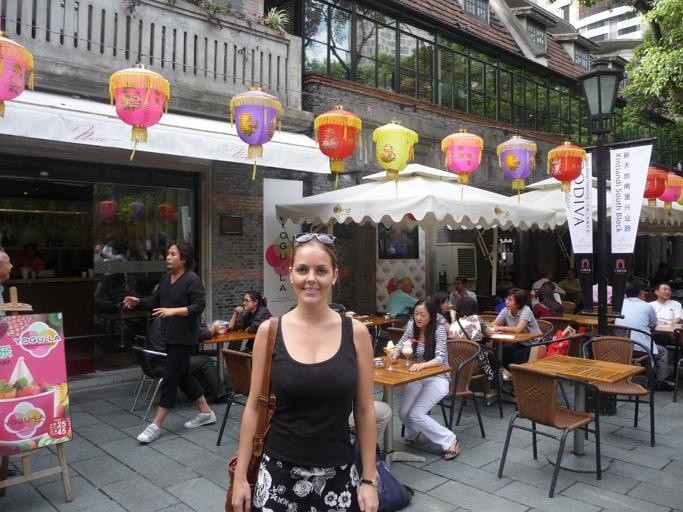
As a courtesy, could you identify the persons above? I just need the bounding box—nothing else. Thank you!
[346,397,393,450]
[384,298,462,462]
[228,232,382,512]
[143,311,232,403]
[93,238,123,297]
[385,278,420,316]
[0,245,19,479]
[436,268,681,396]
[227,289,273,349]
[121,239,218,445]
[9,241,47,277]
[126,240,149,293]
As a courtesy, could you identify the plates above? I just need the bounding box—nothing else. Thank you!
[36,270,56,278]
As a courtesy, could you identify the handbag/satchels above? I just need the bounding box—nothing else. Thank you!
[544,325,576,359]
[353,440,412,512]
[478,350,494,381]
[225,317,279,512]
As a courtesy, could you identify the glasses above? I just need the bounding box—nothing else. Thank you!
[243,298,253,302]
[291,233,339,268]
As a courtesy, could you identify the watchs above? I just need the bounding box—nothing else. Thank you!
[360,479,377,488]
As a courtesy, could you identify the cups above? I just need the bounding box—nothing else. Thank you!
[20,271,28,279]
[81,272,87,277]
[0,387,57,445]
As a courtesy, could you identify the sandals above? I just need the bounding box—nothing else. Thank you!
[443,437,461,460]
[403,431,421,446]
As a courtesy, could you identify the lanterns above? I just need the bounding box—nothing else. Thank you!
[657,171,682,212]
[97,200,120,223]
[311,102,363,190]
[546,140,587,195]
[676,177,683,205]
[127,202,149,225]
[439,127,485,200]
[496,134,539,208]
[157,201,175,223]
[227,83,284,183]
[643,165,667,223]
[371,117,419,191]
[0,29,36,121]
[109,62,170,164]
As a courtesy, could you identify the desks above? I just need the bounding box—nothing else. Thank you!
[518,352,645,473]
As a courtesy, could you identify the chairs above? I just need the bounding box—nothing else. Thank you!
[482,294,683,402]
[498,362,602,497]
[582,335,660,447]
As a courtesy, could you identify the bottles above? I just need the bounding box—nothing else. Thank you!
[45,233,63,248]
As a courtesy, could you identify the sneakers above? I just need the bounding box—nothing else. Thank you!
[183,410,217,429]
[137,422,161,443]
[501,367,513,381]
[654,382,675,391]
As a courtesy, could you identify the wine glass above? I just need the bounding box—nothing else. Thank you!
[401,344,413,366]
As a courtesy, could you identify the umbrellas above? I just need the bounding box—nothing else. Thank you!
[270,159,560,295]
[639,196,683,230]
[507,169,611,227]
